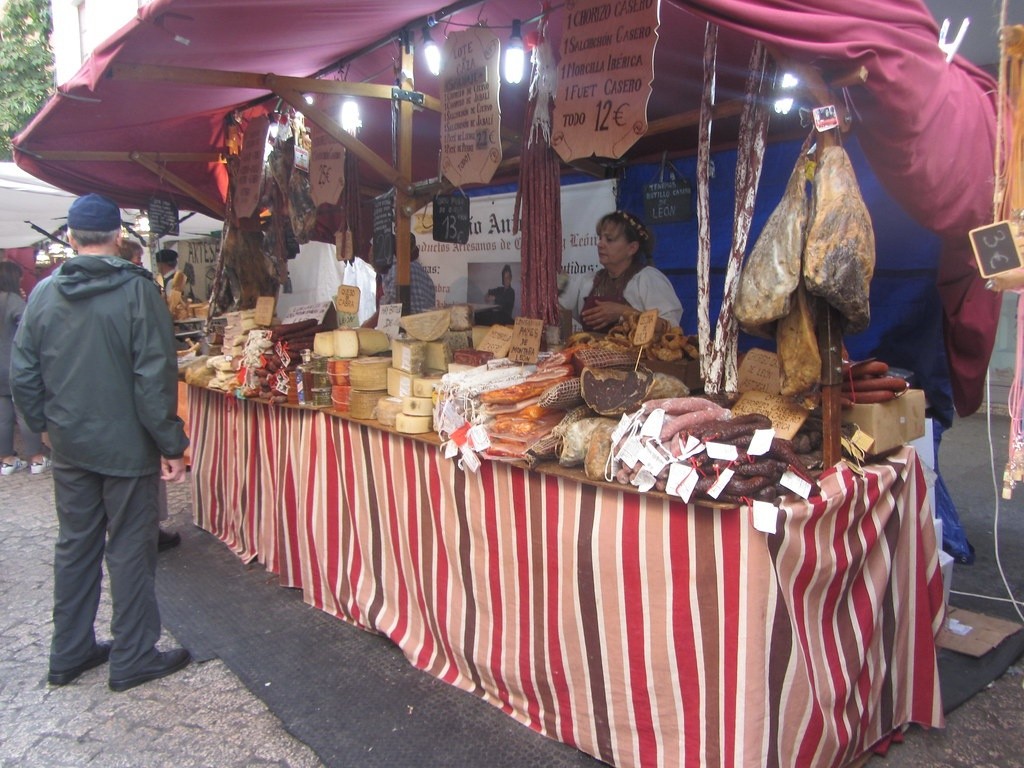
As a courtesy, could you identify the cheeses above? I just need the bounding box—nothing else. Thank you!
[315,303,514,435]
[186,310,270,389]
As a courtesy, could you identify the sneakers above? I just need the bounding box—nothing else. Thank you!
[31,456,51,474]
[1,456,28,475]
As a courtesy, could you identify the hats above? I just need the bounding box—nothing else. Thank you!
[156,249,178,261]
[67,192,120,231]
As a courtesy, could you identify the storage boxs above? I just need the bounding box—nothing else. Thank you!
[843,388,926,456]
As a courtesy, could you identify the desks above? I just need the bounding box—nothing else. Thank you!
[179,370,947,768]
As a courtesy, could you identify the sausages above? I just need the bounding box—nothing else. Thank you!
[821,359,911,408]
[512,94,564,333]
[693,23,782,399]
[612,399,821,504]
[235,319,323,401]
[338,151,362,264]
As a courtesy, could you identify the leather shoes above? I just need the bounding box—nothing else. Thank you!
[48,639,115,685]
[158,524,180,552]
[109,649,191,690]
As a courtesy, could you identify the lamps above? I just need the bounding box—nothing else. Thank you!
[505,19,523,83]
[775,74,798,115]
[421,26,439,76]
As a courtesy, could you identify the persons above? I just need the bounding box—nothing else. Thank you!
[0,193,193,692]
[360,232,436,329]
[578,211,683,334]
[474,265,514,326]
